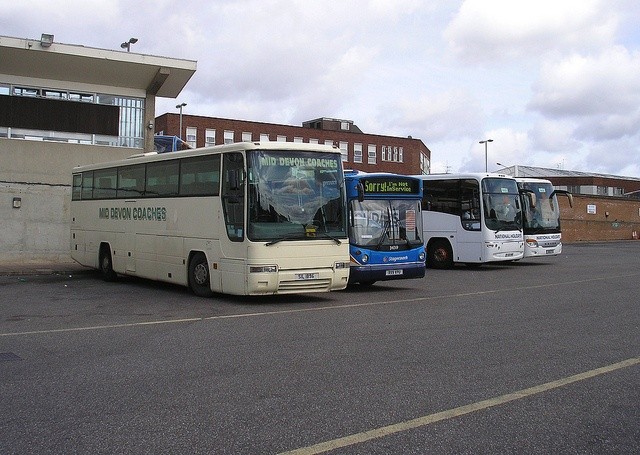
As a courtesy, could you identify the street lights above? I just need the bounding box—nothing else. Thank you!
[176,103,189,140]
[478,139,496,173]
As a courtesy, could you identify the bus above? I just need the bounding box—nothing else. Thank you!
[250,169,432,286]
[408,172,537,270]
[515,177,573,258]
[69,140,364,297]
